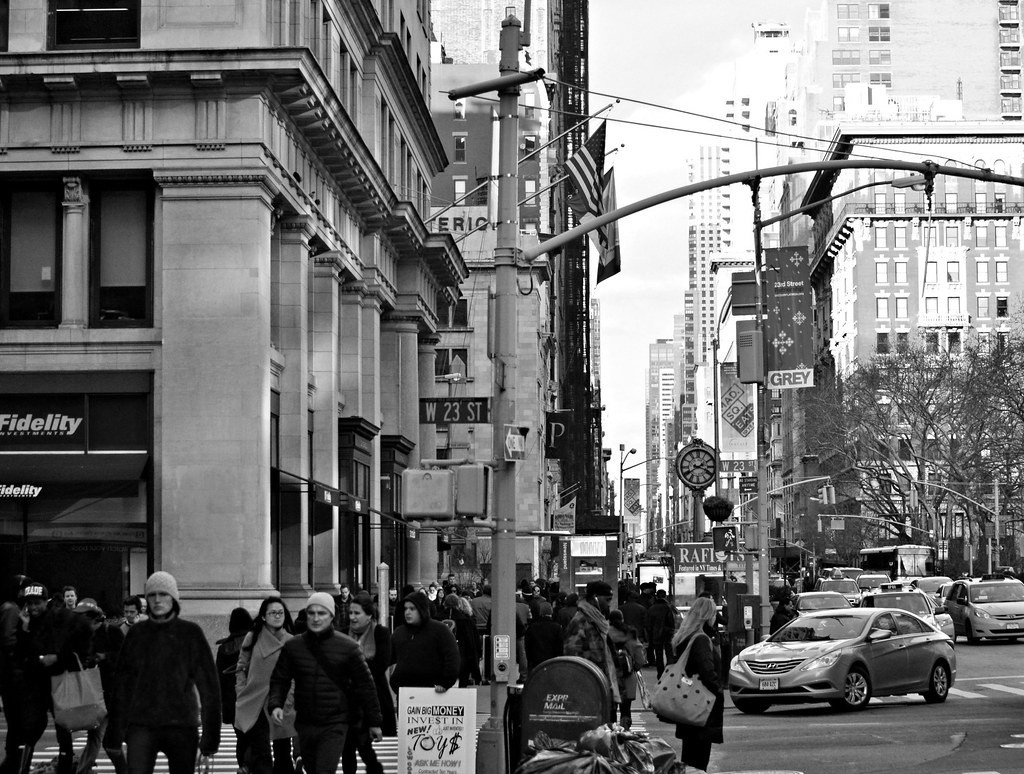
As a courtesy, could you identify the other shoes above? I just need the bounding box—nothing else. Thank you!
[482,679,490,686]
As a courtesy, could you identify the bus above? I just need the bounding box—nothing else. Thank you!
[860,545,938,593]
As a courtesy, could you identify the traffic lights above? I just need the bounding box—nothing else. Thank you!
[905,491,914,509]
[818,487,828,505]
[711,526,740,553]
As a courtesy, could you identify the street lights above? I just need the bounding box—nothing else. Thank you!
[619,442,638,581]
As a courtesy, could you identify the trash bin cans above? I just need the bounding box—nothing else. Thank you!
[523,656,610,756]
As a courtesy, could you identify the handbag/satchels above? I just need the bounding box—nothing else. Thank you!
[647,633,716,725]
[51,652,107,729]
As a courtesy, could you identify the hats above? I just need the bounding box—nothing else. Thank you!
[306,591,335,617]
[586,581,613,601]
[24,582,48,603]
[72,597,103,616]
[145,571,179,603]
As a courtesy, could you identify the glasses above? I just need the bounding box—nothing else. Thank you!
[265,610,284,616]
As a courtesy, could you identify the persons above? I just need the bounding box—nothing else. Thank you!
[638,581,682,682]
[1,573,150,774]
[769,599,793,634]
[120,571,221,774]
[332,581,482,736]
[671,596,725,771]
[699,592,729,658]
[215,591,384,774]
[559,577,648,731]
[443,571,562,685]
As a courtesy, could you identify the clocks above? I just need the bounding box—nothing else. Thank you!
[675,437,716,491]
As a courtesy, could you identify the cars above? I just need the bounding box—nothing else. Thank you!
[726,604,958,717]
[769,565,961,650]
[942,572,1024,646]
